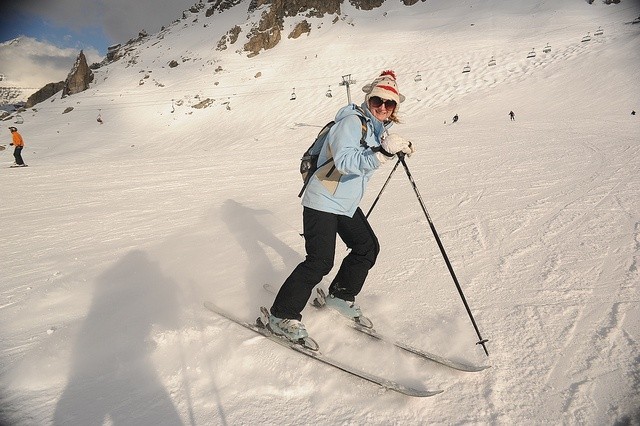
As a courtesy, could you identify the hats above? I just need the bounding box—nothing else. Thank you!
[363,70,406,115]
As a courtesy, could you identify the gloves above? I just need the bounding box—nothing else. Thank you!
[374,133,413,163]
[10,143,14,146]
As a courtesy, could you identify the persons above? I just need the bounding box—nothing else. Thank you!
[8,127,24,166]
[269,70,414,344]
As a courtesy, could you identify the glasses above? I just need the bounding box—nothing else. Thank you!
[368,96,396,110]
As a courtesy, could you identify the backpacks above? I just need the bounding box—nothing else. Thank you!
[299,104,368,181]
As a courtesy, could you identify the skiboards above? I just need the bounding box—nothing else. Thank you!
[201,283,492,399]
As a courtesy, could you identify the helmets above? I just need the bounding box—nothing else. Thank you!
[9,127,17,132]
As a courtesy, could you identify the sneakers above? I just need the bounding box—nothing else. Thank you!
[324,294,362,321]
[269,314,308,343]
